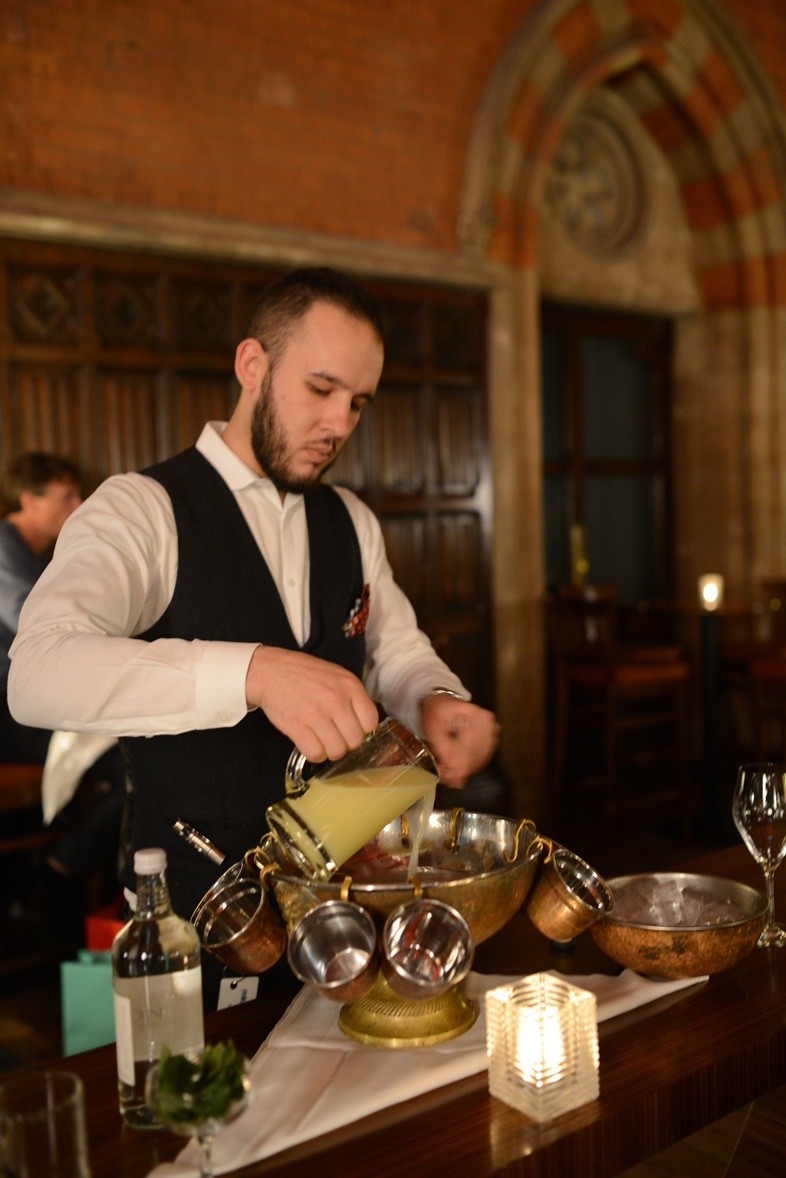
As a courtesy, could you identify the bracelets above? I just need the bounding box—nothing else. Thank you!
[429,688,463,699]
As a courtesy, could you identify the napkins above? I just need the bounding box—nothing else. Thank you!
[152,957,711,1177]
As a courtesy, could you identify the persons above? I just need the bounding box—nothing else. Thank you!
[0,449,82,764]
[7,262,499,953]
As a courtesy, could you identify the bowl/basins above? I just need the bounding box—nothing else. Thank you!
[589,871,771,981]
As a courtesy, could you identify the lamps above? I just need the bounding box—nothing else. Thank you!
[483,972,600,1122]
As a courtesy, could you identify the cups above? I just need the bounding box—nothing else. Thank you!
[379,879,475,1001]
[0,1072,90,1177]
[186,860,289,978]
[524,835,616,945]
[285,875,382,1006]
[264,714,442,882]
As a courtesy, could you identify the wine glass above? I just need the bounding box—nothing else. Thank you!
[732,760,786,951]
[147,1051,251,1178]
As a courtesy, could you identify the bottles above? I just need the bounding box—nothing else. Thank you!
[109,847,207,1133]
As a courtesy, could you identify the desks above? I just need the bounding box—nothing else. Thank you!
[0,809,786,1177]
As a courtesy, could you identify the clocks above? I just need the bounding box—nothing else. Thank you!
[535,108,644,263]
